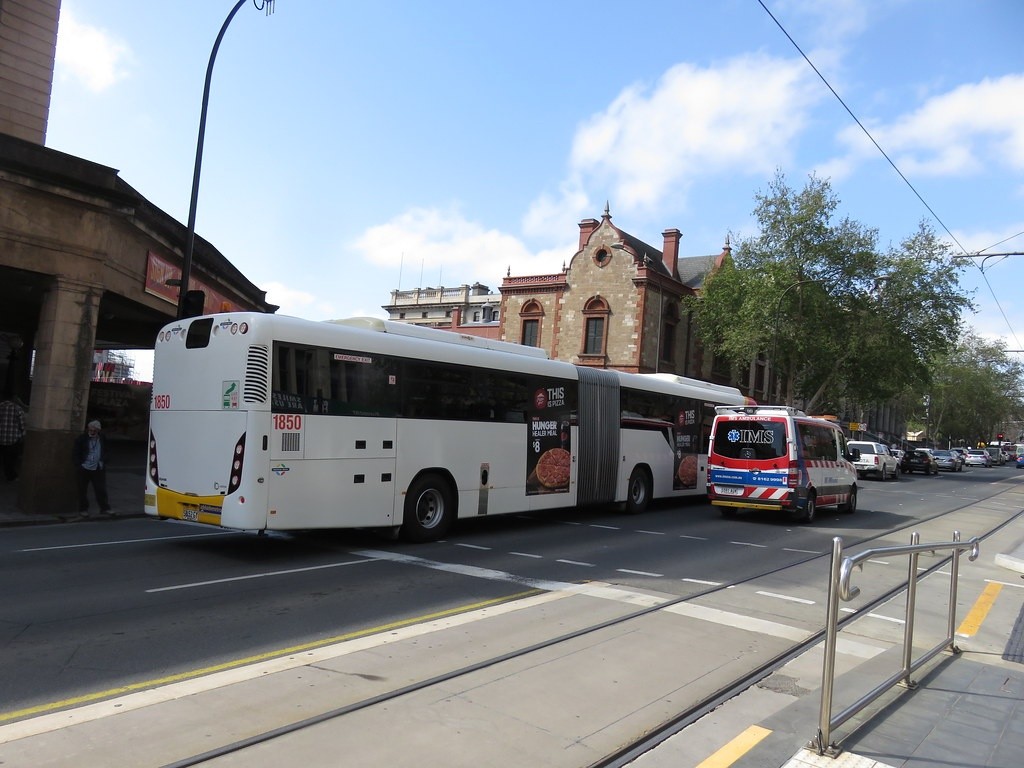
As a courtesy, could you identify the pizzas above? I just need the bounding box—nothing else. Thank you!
[536,446,570,489]
[679,457,698,485]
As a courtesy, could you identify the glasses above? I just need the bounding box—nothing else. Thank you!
[89,429,97,432]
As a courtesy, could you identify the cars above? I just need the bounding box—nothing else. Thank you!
[888,449,906,467]
[899,442,1024,476]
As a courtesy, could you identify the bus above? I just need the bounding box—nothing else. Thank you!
[142,315,760,545]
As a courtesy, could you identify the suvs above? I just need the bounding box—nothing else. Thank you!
[845,441,899,481]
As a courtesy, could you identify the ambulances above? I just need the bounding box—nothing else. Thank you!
[706,404,857,521]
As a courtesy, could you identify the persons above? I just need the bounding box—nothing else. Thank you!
[73,420,116,517]
[0,390,27,481]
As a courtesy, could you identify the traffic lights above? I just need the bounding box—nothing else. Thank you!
[183,290,205,317]
[998,434,1003,438]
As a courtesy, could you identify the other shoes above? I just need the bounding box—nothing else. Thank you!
[80,513,90,518]
[101,511,121,516]
